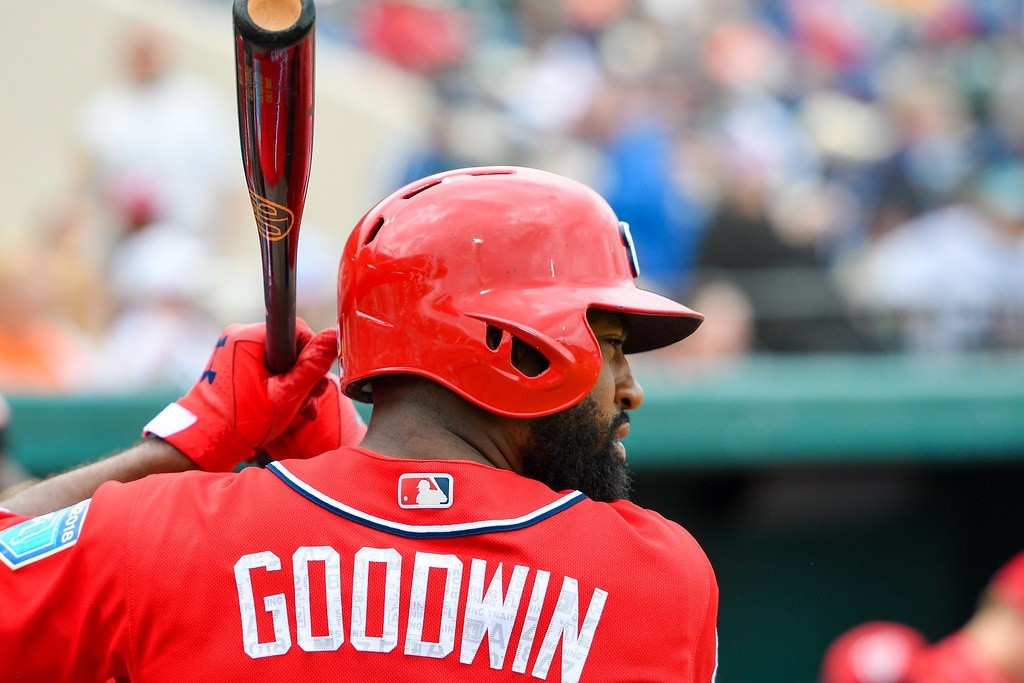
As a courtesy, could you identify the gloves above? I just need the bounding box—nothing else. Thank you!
[259,373,369,460]
[142,316,339,474]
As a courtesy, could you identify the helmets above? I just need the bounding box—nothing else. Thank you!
[337,164,705,420]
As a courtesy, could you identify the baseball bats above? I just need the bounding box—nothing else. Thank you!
[231,0,317,375]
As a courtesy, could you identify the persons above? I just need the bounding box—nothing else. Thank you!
[1,166,718,683]
[0,1,1024,388]
